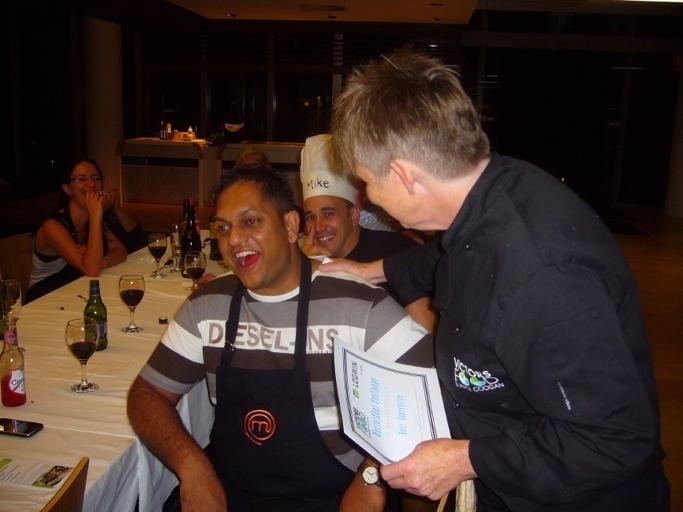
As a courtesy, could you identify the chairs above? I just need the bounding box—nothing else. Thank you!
[40,455,90,512]
[1,232,33,303]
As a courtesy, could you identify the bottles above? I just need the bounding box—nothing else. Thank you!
[181,194,203,280]
[0,318,25,407]
[158,108,211,142]
[83,279,108,352]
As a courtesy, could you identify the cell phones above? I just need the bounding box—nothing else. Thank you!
[0,418,44,437]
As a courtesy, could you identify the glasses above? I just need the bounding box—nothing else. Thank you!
[70,175,101,184]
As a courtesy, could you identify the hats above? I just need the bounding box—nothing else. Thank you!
[299,134,358,205]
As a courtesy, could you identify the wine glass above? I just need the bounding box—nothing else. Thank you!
[118,274,145,335]
[183,251,206,295]
[0,279,25,354]
[148,233,168,279]
[64,318,100,394]
[170,223,180,273]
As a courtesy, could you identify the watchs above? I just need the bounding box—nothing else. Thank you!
[355,462,389,491]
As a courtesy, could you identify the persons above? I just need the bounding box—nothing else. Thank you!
[99,187,148,258]
[128,165,441,511]
[19,158,129,308]
[296,194,434,331]
[306,50,677,511]
[356,184,426,246]
